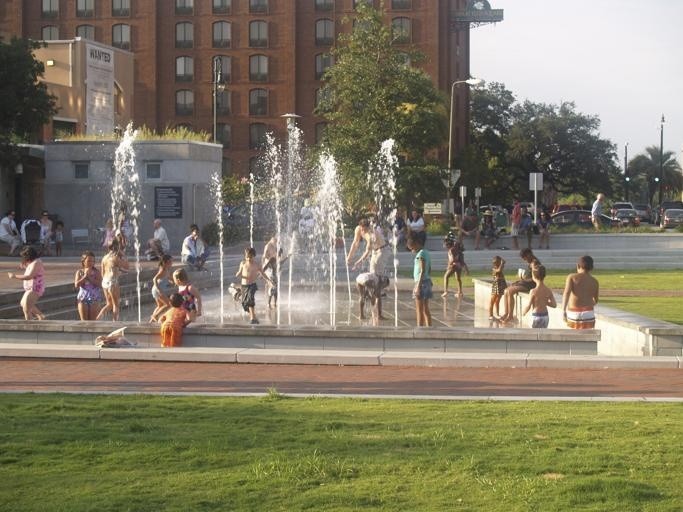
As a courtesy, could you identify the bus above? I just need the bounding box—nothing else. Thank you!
[477,200,682,230]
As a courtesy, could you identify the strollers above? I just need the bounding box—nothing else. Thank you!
[19,218,46,257]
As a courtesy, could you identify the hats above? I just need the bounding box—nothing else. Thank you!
[482,210,493,216]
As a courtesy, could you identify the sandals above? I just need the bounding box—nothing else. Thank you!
[250,320,259,324]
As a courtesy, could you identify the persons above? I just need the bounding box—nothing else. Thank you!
[39,212,54,257]
[349,197,557,328]
[228,232,291,323]
[298,215,314,253]
[55,221,64,256]
[591,193,605,231]
[0,209,22,257]
[562,255,599,329]
[7,246,46,320]
[74,198,208,348]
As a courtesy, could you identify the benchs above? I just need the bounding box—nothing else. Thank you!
[0,240,56,256]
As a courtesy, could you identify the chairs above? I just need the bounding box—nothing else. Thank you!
[71,228,93,255]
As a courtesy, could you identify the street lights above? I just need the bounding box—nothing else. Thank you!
[657,112,665,203]
[212,54,226,143]
[624,140,630,201]
[444,78,484,218]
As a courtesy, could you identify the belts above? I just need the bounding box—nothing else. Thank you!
[374,242,390,252]
